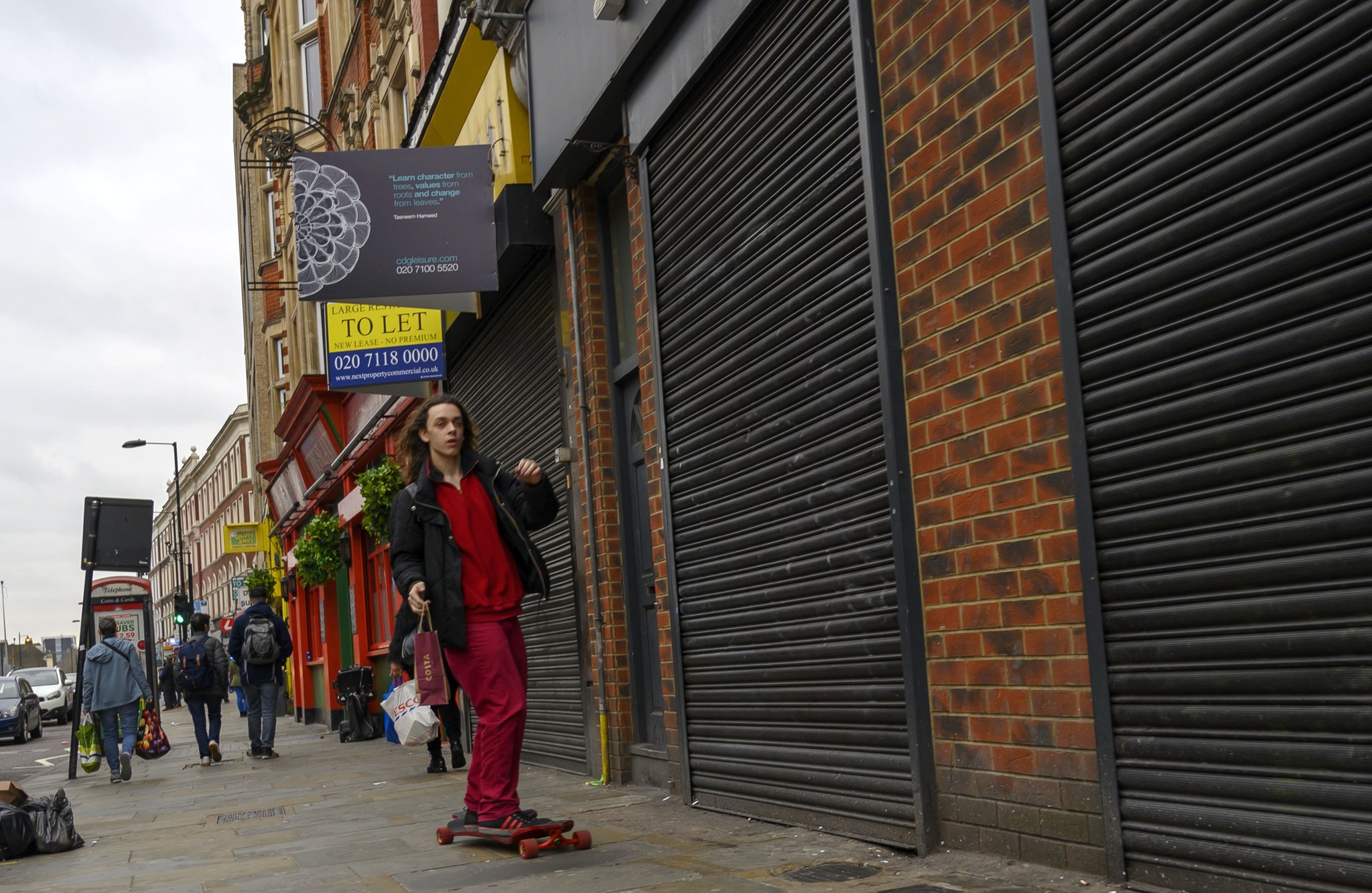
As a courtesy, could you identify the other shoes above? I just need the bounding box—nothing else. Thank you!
[209,740,222,762]
[120,753,132,781]
[240,711,247,718]
[202,756,211,766]
[174,702,181,708]
[110,771,121,783]
[162,707,171,711]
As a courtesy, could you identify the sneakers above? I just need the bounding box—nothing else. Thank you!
[262,749,279,759]
[247,748,262,757]
[464,805,537,828]
[478,811,537,836]
[449,737,466,769]
[427,756,448,773]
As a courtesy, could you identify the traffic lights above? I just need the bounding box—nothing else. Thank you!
[173,593,188,624]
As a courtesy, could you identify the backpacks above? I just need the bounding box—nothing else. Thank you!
[180,636,216,691]
[242,612,286,666]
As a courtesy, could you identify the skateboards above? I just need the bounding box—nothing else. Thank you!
[436,811,591,858]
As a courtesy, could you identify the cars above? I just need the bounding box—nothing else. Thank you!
[0,676,43,744]
[64,673,76,694]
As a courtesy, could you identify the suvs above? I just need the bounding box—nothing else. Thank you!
[0,665,75,726]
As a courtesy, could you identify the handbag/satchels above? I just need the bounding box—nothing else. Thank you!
[75,712,101,773]
[380,671,442,746]
[136,703,171,760]
[413,602,448,705]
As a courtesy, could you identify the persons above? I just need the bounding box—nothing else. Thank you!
[82,616,153,782]
[228,658,248,717]
[227,585,293,758]
[158,656,174,711]
[388,395,558,832]
[172,613,228,766]
[171,654,182,707]
[388,599,466,772]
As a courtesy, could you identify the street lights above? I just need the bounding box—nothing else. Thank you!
[19,631,30,666]
[122,438,187,643]
